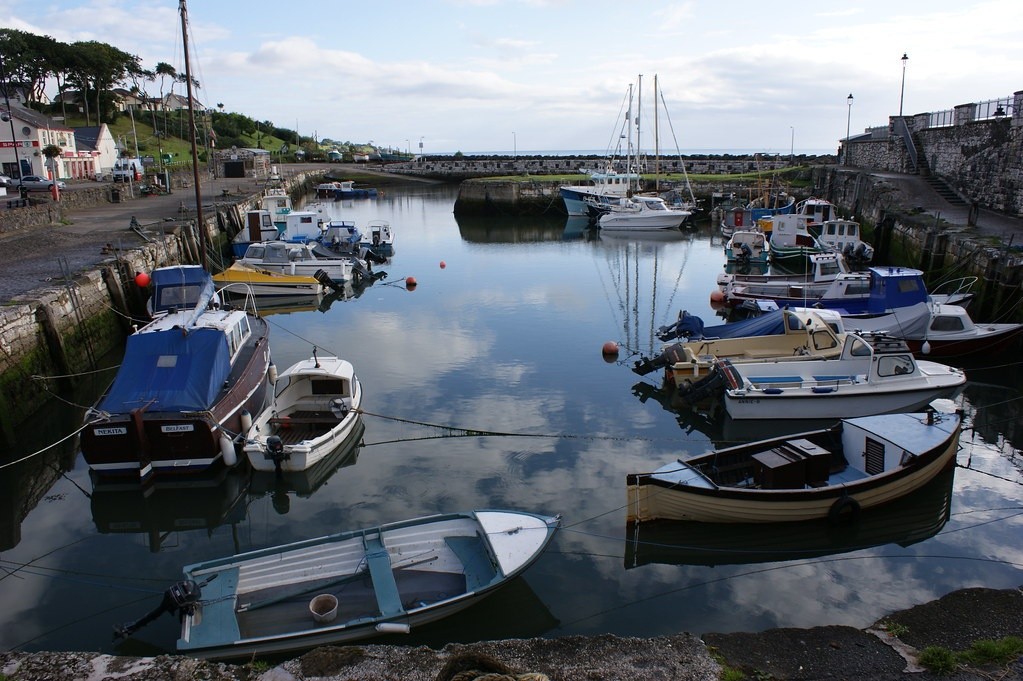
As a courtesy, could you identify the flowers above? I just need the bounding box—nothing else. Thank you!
[120,149,133,159]
[43,144,63,159]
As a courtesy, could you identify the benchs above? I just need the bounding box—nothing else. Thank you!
[363,529,404,617]
[747,376,856,387]
[744,350,799,360]
[267,417,343,431]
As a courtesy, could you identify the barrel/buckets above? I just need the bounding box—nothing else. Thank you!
[309,594,338,623]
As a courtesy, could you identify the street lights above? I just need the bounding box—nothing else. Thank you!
[846,94,853,140]
[420,137,425,154]
[900,52,909,115]
[511,131,517,156]
[406,140,410,152]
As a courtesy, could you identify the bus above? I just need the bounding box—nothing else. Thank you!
[294,150,305,162]
[328,151,340,163]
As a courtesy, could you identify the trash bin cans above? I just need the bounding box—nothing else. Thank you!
[111,189,121,203]
[162,154,172,165]
[18,187,28,198]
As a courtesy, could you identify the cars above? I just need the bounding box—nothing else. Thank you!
[0,171,66,193]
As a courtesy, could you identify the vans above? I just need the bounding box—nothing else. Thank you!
[111,159,141,183]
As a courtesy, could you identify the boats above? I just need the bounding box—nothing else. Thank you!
[244,344,363,474]
[81,257,272,484]
[626,409,961,544]
[210,165,393,312]
[661,310,865,389]
[718,330,967,420]
[656,153,1023,357]
[176,508,562,667]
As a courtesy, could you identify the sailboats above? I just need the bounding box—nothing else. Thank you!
[559,74,703,233]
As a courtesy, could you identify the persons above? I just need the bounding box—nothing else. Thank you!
[837,146,842,164]
[374,235,380,252]
[736,242,752,264]
[331,235,340,252]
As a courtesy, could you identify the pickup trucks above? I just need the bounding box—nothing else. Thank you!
[352,153,369,163]
